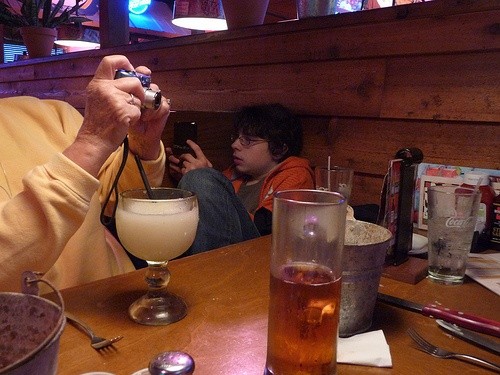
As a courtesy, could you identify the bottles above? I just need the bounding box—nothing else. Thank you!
[147,351,195,375]
[455,171,494,253]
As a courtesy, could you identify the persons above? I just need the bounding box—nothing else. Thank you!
[0,55,172,296]
[128,103,316,269]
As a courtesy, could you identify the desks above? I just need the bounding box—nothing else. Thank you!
[34,221,500,375]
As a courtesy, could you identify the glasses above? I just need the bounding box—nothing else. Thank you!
[230,132,268,147]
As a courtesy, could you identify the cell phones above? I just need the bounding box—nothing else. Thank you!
[173,121,199,169]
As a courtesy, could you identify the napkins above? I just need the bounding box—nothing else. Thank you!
[337,329,393,368]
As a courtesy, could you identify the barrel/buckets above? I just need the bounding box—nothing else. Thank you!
[338,219,392,338]
[0,271,66,374]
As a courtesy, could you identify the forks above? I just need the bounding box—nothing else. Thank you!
[65,314,123,351]
[408,328,500,372]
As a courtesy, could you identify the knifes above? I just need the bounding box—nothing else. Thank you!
[376,292,500,336]
[436,319,500,355]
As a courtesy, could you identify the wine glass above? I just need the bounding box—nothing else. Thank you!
[116,187,201,326]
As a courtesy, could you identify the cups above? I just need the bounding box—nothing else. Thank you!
[316,166,354,206]
[427,186,482,283]
[263,190,348,375]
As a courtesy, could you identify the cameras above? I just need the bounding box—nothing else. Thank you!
[114,69,161,110]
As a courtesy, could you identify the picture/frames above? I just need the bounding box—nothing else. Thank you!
[417,173,482,233]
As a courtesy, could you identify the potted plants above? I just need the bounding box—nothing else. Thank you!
[0,0,92,59]
